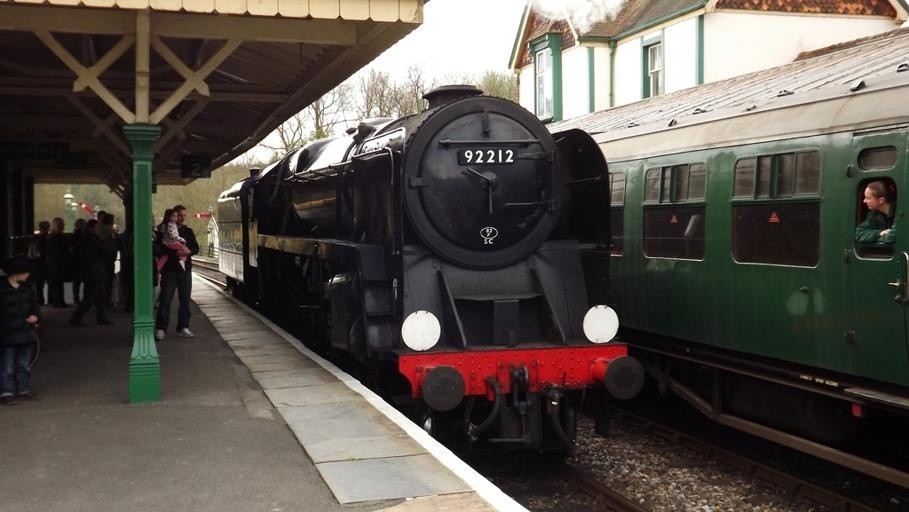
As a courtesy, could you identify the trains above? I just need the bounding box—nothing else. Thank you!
[547,29,906,489]
[211,81,643,473]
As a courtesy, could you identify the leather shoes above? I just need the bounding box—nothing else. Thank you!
[69,316,115,327]
[1,390,42,405]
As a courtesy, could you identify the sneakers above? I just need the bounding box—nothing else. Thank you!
[156,327,194,340]
[179,260,186,270]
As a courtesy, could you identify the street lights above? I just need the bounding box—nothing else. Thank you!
[71,202,78,214]
[62,190,73,220]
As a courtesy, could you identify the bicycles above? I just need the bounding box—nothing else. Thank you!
[30,322,44,372]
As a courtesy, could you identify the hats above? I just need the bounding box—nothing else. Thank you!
[6,255,35,273]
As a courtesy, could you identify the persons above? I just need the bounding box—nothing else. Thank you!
[2,255,41,405]
[854,181,897,245]
[33,209,134,329]
[154,209,190,277]
[152,204,199,340]
